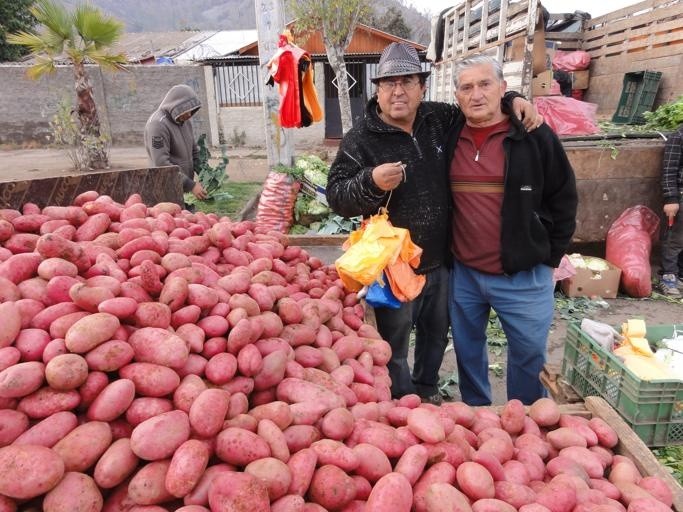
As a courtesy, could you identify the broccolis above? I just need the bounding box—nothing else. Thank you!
[297,155,330,214]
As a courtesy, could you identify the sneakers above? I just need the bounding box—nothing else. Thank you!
[658,273,682,299]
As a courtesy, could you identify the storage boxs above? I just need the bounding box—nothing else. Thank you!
[562,255,621,299]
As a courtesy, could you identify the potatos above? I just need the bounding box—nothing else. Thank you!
[0,190,675,512]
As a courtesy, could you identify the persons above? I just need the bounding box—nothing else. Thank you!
[445,54,578,407]
[327,42,545,406]
[144,84,208,213]
[657,125,683,296]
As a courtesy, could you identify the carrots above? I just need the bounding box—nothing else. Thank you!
[255,171,300,235]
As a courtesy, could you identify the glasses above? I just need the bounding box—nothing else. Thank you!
[380,79,420,90]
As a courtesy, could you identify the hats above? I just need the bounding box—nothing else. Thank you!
[370,43,431,84]
